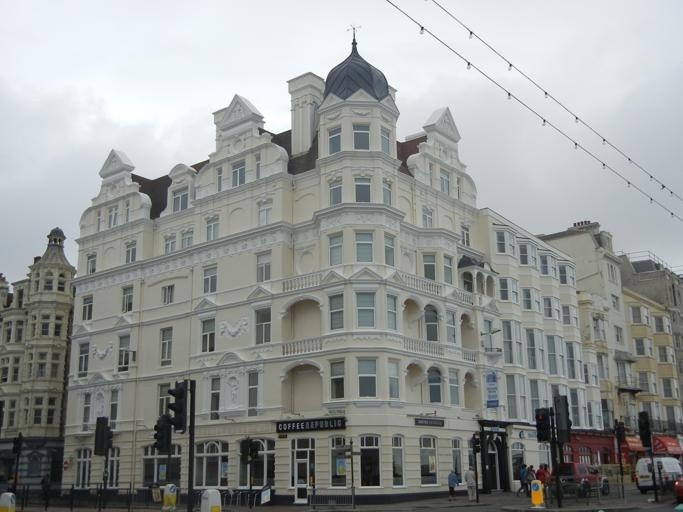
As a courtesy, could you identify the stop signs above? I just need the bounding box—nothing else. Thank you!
[63,459,70,471]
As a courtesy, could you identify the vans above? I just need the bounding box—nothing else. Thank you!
[636,457,683,494]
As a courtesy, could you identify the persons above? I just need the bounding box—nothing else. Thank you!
[447,469,460,503]
[40,473,49,500]
[7,476,14,491]
[516,463,551,497]
[463,465,477,501]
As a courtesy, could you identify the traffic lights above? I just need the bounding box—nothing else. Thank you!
[12,437,23,455]
[617,421,625,444]
[95,417,113,453]
[534,408,552,442]
[638,411,651,447]
[472,435,481,454]
[167,378,188,431]
[153,414,171,453]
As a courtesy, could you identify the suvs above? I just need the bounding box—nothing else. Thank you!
[550,463,610,497]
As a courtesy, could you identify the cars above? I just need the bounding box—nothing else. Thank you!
[673,474,683,502]
